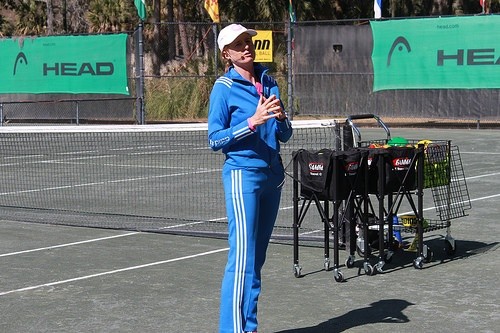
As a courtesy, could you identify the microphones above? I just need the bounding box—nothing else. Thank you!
[235,56,244,62]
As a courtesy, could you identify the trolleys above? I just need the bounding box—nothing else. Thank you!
[347,114,472,263]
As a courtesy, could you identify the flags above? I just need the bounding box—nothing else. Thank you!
[374,0,381,18]
[135,0,146,24]
[205,0,220,23]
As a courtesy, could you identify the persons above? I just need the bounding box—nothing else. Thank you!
[208,23,293,333]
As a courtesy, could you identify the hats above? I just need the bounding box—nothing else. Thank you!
[217,23,257,52]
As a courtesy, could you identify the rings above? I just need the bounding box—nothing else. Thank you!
[266,111,269,114]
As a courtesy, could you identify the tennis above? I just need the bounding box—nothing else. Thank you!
[414,140,451,187]
